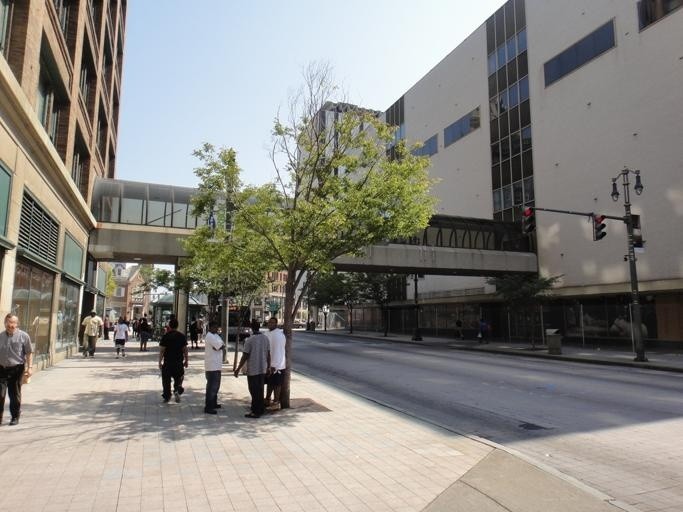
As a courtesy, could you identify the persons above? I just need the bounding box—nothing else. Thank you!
[197,313,204,343]
[31,300,78,371]
[265,317,287,411]
[104,315,169,359]
[456,319,464,340]
[234,320,271,418]
[82,309,104,358]
[204,321,226,415]
[159,319,188,404]
[477,319,490,345]
[0,312,34,426]
[189,317,200,350]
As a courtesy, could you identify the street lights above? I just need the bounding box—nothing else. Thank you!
[610,165,648,362]
[306,270,311,330]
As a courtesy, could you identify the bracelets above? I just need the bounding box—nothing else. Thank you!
[28,367,32,368]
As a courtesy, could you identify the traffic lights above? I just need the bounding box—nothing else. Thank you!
[592,212,606,241]
[522,205,535,235]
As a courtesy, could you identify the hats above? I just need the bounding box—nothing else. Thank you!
[90,309,96,314]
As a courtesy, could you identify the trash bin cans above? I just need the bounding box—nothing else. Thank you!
[546,329,563,354]
[310,321,315,331]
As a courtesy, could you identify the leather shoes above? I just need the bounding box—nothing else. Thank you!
[244,412,261,418]
[9,418,19,424]
[204,404,221,414]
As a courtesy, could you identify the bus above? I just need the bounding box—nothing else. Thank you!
[419,308,482,330]
[205,305,251,341]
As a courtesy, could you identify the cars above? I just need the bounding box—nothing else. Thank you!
[277,319,306,329]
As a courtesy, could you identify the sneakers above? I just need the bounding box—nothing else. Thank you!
[84,354,95,359]
[264,398,281,411]
[174,392,181,403]
[163,398,169,403]
[115,354,126,359]
